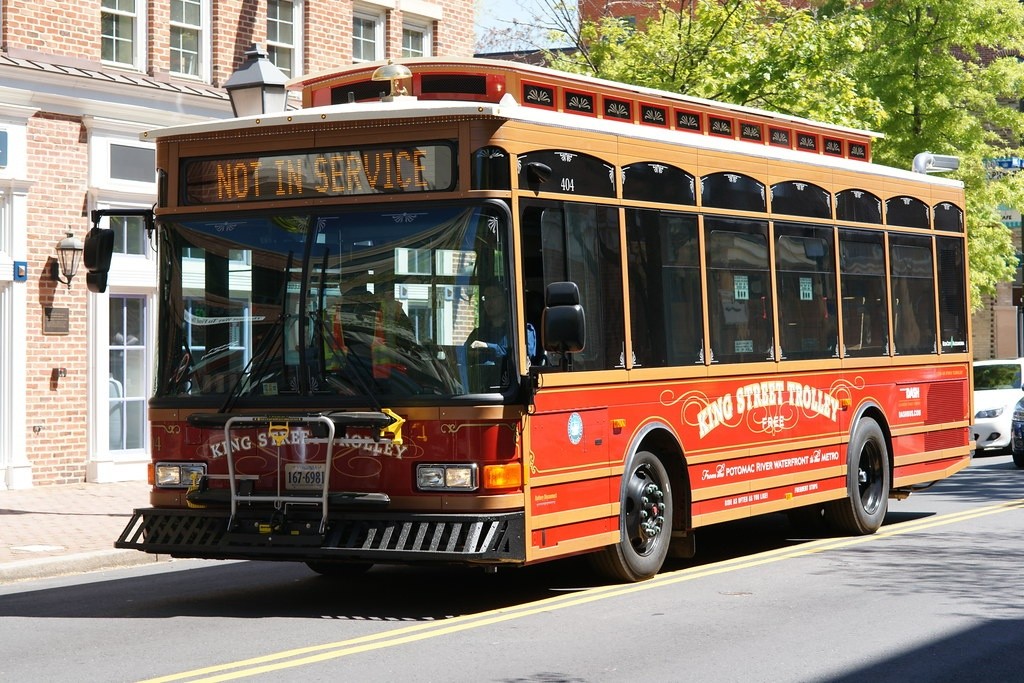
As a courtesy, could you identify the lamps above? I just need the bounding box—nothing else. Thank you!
[49,232,83,290]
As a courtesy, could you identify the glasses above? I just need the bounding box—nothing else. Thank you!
[481,294,504,301]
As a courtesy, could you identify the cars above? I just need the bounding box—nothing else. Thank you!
[972,359,1024,454]
[1011,383,1024,468]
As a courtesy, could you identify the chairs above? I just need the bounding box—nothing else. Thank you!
[842,294,899,350]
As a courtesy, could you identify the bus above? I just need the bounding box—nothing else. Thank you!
[84,54,976,583]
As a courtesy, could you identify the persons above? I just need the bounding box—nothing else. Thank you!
[464,283,537,392]
[315,259,424,396]
